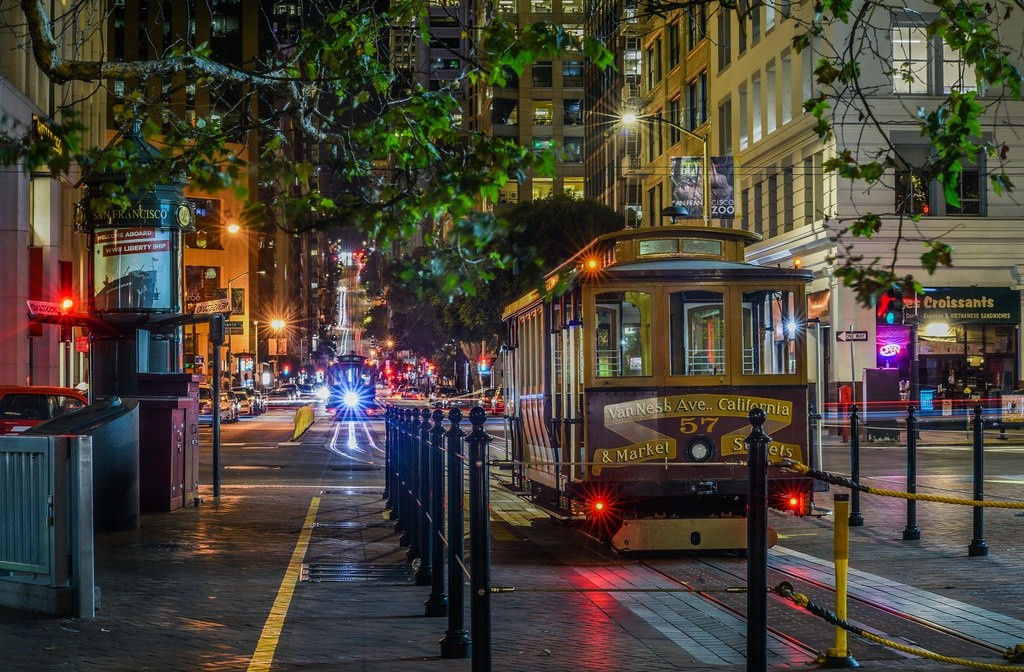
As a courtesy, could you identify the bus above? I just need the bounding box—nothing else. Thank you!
[501,205,816,553]
[318,350,378,418]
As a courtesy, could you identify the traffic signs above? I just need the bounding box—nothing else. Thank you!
[836,330,869,342]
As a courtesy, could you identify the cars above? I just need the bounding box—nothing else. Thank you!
[198,381,315,424]
[0,384,90,435]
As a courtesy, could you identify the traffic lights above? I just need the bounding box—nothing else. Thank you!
[885,286,905,325]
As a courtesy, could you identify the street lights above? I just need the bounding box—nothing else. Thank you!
[270,319,285,387]
[300,334,317,386]
[622,111,713,227]
[226,269,267,390]
